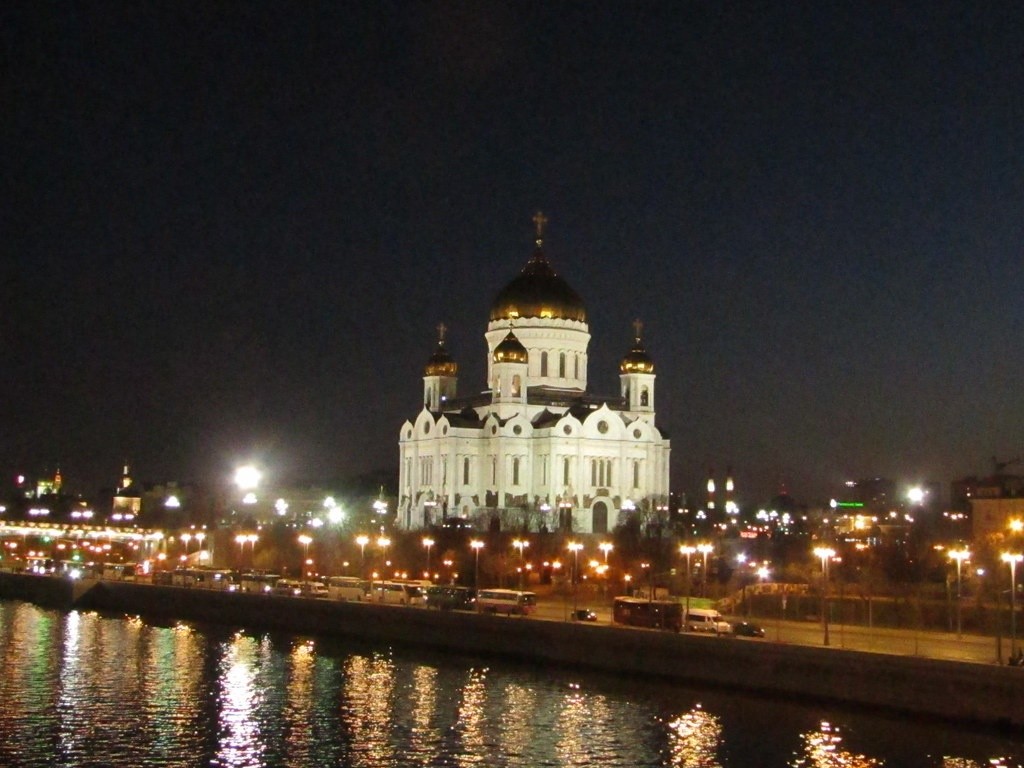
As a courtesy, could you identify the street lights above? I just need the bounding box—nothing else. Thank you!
[423,539,437,588]
[1,530,370,578]
[469,542,486,593]
[511,540,530,590]
[999,551,1024,667]
[812,547,835,643]
[697,544,714,599]
[599,541,616,605]
[947,549,970,638]
[377,535,391,586]
[680,546,695,623]
[566,542,583,621]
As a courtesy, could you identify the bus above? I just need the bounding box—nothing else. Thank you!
[477,588,538,617]
[611,596,684,631]
[17,557,430,610]
[428,587,468,610]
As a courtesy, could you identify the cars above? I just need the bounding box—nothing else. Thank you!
[735,620,765,638]
[571,608,598,621]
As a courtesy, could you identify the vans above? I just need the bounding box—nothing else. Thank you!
[684,606,731,635]
